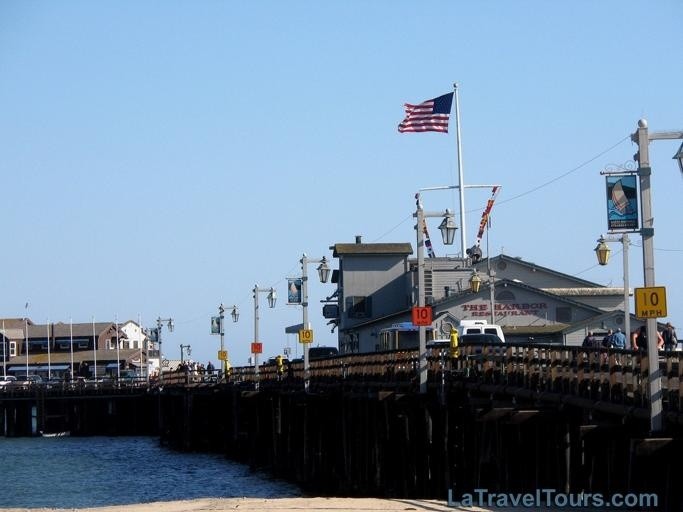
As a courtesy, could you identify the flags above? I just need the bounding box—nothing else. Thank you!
[398,91,455,133]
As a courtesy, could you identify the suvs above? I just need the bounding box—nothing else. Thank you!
[262,344,339,369]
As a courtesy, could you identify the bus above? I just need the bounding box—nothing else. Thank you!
[378,321,436,351]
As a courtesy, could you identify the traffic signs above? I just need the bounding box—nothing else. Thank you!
[217,351,227,360]
[412,306,433,327]
[632,286,667,319]
[299,329,314,343]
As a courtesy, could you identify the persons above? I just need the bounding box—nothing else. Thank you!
[180,360,215,381]
[582,321,678,365]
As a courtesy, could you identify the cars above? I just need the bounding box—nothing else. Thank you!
[426,338,449,346]
[460,334,509,357]
[0,370,137,391]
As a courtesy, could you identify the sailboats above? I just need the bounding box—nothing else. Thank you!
[611,179,637,218]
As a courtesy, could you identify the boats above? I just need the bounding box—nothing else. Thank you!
[38,430,71,439]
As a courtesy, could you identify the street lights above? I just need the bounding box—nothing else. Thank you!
[252,283,278,377]
[630,117,683,438]
[218,303,240,380]
[180,343,193,369]
[471,268,498,326]
[593,231,632,367]
[411,190,459,401]
[301,251,333,372]
[157,315,176,387]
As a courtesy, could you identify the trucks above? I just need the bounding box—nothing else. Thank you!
[454,318,506,344]
